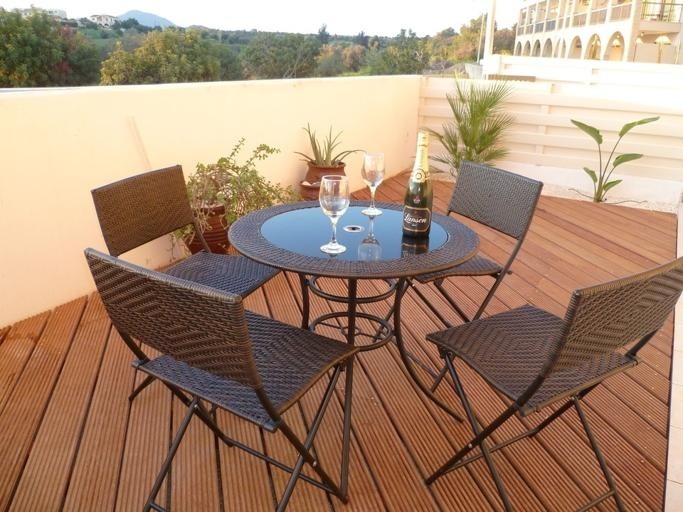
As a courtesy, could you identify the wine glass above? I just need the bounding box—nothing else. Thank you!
[357,213,383,260]
[359,152,386,217]
[318,174,351,255]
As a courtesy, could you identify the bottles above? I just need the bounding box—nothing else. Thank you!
[400,129,432,239]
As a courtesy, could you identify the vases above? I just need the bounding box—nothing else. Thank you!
[297,179,336,201]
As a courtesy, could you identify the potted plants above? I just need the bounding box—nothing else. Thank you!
[293,121,368,181]
[166,136,305,256]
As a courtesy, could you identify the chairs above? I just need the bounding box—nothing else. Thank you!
[90,163,285,448]
[371,160,545,394]
[81,247,361,512]
[424,256,683,512]
